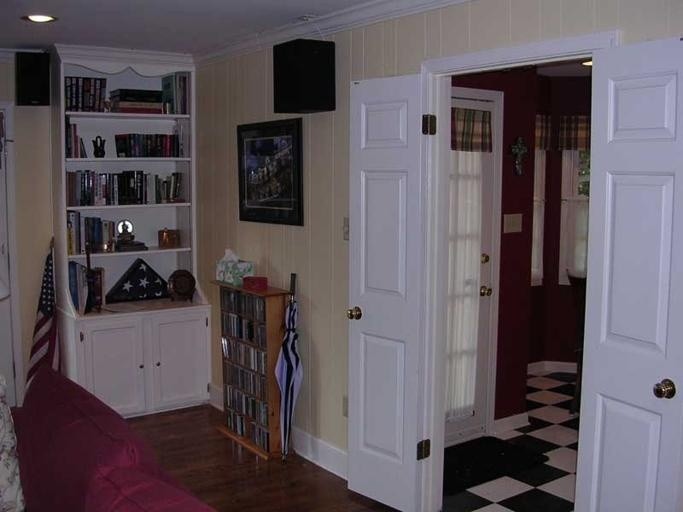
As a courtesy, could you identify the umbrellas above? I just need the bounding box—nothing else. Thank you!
[273,272,305,462]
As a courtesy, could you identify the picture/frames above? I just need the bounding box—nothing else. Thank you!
[234,117,305,228]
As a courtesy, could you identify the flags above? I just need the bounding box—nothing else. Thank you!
[24,236,60,386]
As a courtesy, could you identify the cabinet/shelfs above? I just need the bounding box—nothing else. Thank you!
[207,277,290,460]
[46,44,212,422]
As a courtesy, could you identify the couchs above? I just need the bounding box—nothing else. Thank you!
[0,365,219,511]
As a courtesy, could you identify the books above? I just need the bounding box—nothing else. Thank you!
[63,72,189,253]
[217,285,270,455]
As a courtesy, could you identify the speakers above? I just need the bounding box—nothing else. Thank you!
[15,51,50,106]
[273,38,336,114]
